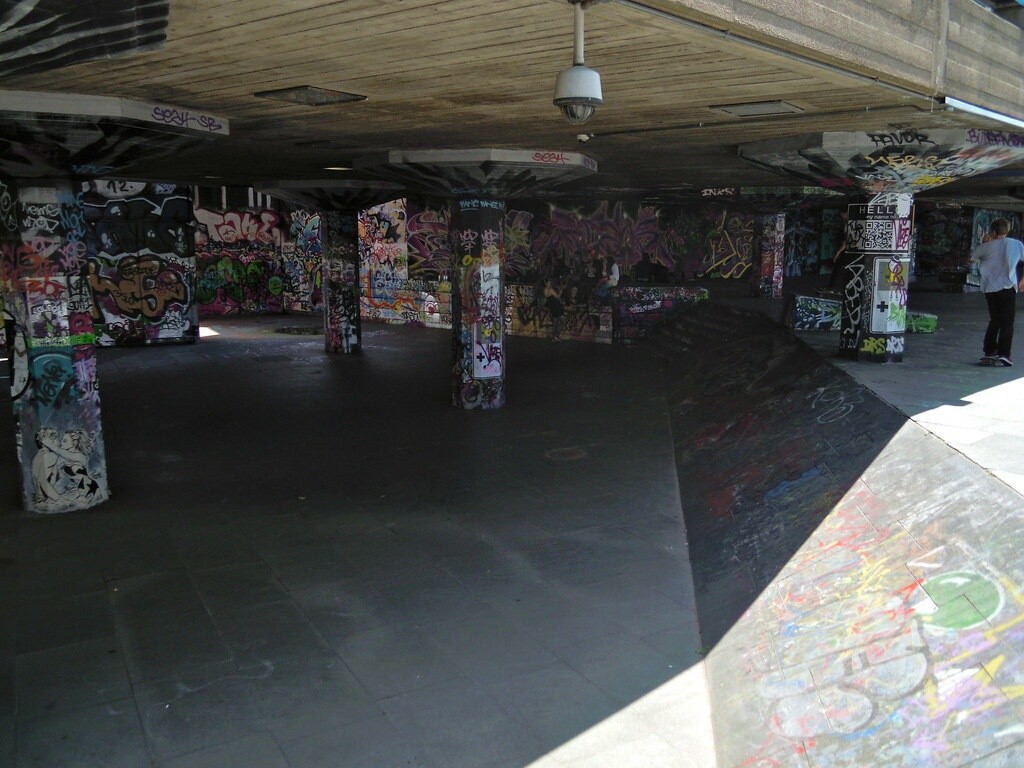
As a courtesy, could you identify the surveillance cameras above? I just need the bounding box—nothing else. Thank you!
[577,134,589,143]
[552,66,602,127]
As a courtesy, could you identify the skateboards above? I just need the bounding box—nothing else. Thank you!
[979,356,1014,367]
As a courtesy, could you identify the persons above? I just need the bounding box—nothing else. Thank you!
[973,218,1024,364]
[544,253,620,341]
[818,206,848,296]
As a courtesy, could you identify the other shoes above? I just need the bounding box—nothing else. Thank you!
[554,337,562,343]
[998,355,1014,366]
[985,350,1000,358]
[552,339,556,343]
